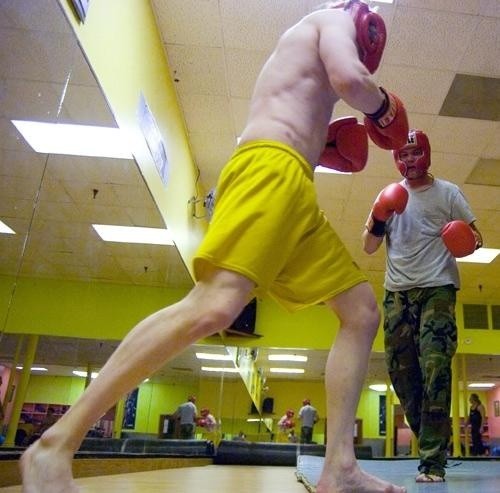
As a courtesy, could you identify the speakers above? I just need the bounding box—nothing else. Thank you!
[262,397,273,413]
[227,297,257,334]
[251,402,259,415]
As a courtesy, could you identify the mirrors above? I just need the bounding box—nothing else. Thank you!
[263,378,500,452]
[2,0,263,455]
[0,332,264,455]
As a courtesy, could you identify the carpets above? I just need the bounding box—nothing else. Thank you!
[295,454,500,492]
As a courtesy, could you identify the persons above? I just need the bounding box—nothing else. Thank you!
[297,400,318,444]
[20,0,409,493]
[462,394,486,458]
[363,130,481,483]
[278,409,294,443]
[174,396,197,440]
[198,409,216,442]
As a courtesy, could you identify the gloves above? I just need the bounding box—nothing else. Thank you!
[365,183,408,236]
[441,220,482,257]
[320,86,408,172]
[197,419,206,425]
[284,420,292,428]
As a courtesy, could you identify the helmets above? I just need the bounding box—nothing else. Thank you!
[331,0,386,75]
[188,395,196,403]
[303,399,311,405]
[201,408,209,416]
[392,129,430,180]
[287,410,294,418]
